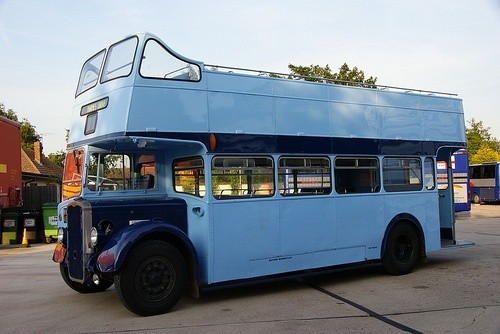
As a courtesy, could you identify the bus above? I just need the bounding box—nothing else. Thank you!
[47,30,477,319]
[468,161,500,206]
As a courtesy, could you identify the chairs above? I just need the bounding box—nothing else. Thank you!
[139,174,154,188]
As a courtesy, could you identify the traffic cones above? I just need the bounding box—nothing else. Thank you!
[22,227,29,247]
